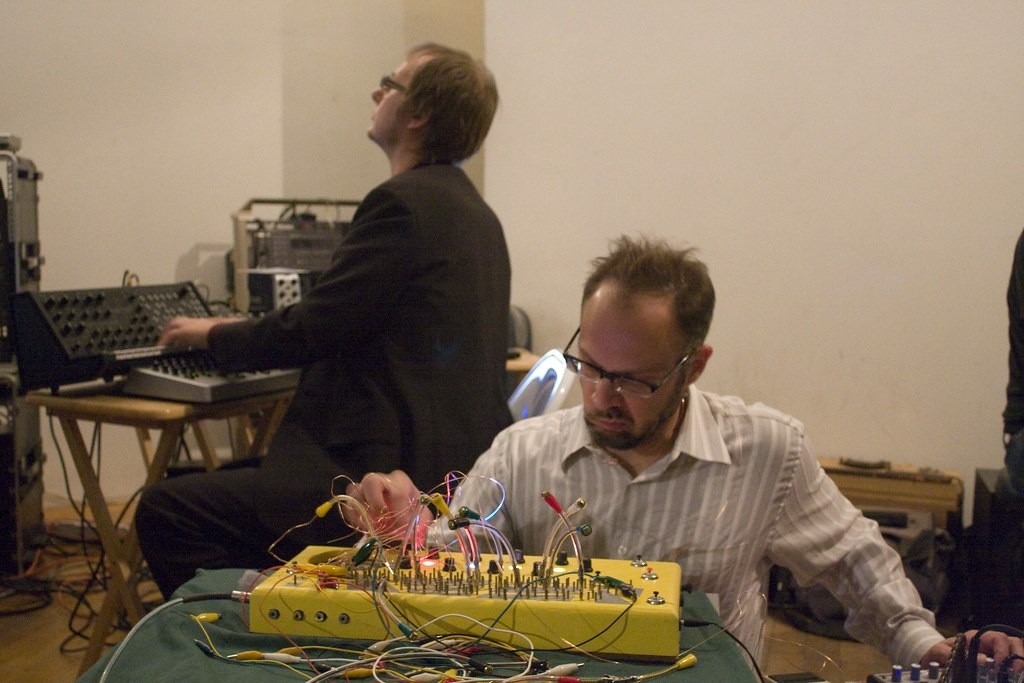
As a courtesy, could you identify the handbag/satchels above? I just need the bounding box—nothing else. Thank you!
[781,507,945,637]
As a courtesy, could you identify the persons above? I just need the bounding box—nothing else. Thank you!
[136,44,514,600]
[343,241,1024,674]
[1004,229,1024,493]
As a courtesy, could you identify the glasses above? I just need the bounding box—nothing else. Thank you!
[380,76,406,92]
[563,327,691,398]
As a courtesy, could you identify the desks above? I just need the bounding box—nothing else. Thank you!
[823,472,961,530]
[82,568,761,683]
[45,347,541,679]
